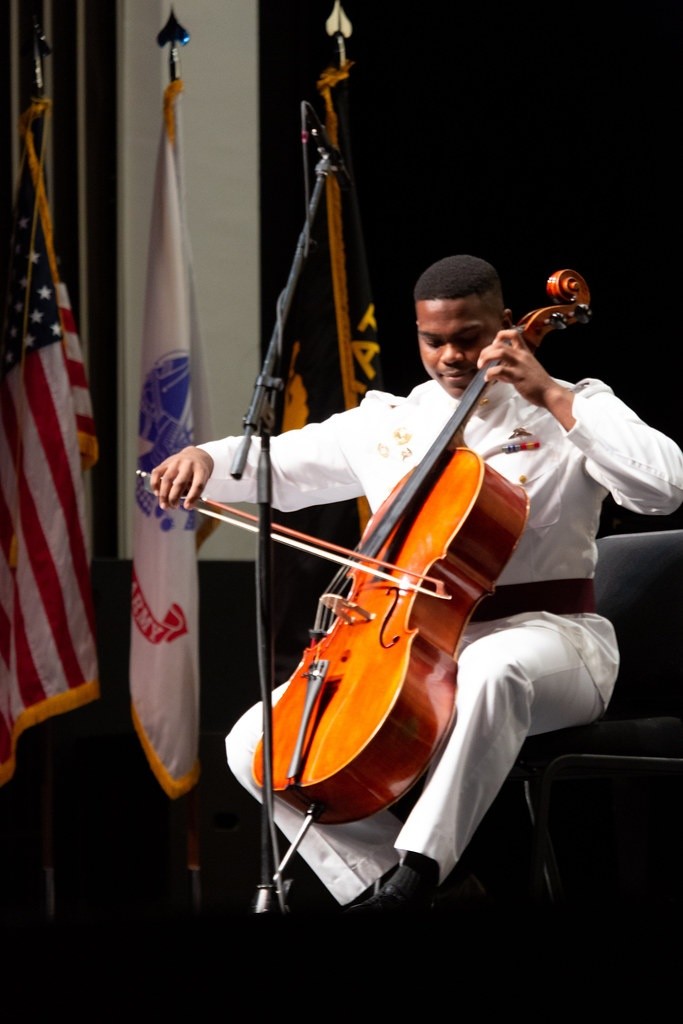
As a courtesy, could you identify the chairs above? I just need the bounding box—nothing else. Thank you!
[508,528,683,933]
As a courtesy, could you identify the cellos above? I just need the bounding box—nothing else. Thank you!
[248,267,595,887]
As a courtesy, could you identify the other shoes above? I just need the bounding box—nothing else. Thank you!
[323,865,433,936]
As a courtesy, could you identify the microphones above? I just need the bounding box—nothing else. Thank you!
[305,102,354,190]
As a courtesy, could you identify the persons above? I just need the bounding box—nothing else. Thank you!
[149,254,683,932]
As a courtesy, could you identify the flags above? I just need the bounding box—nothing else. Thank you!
[130,83,219,794]
[281,74,383,431]
[0,101,100,787]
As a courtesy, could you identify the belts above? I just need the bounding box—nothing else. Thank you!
[468,578,595,623]
